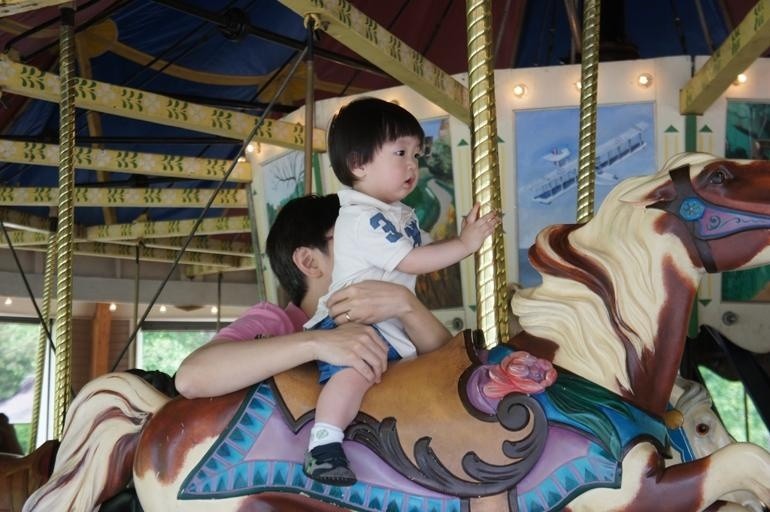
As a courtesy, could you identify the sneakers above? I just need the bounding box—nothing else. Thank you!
[302,441,357,486]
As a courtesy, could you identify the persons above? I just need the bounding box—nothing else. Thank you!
[302,97,501,485]
[169,194,453,399]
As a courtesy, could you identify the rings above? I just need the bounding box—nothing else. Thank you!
[346,313,352,321]
